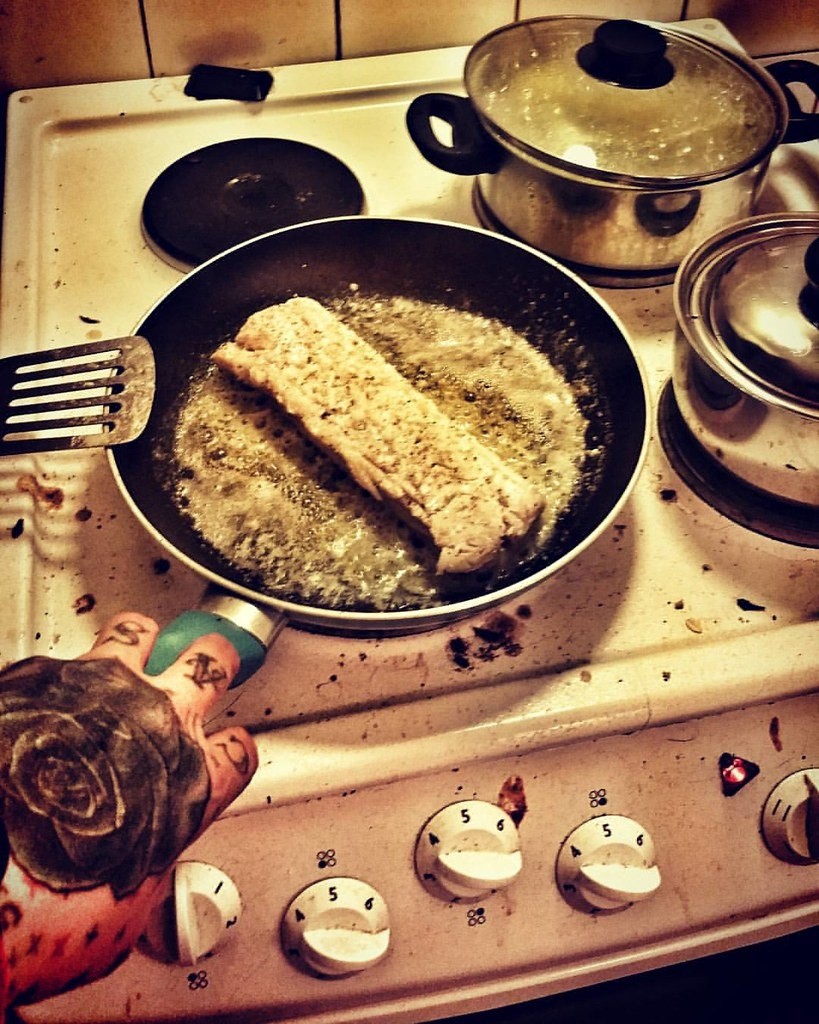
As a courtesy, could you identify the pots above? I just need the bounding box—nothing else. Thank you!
[405,13,818,272]
[671,213,817,505]
[104,216,653,698]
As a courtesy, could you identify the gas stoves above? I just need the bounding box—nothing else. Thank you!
[9,21,815,1020]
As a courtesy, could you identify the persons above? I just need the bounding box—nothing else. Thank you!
[0,609,259,1007]
